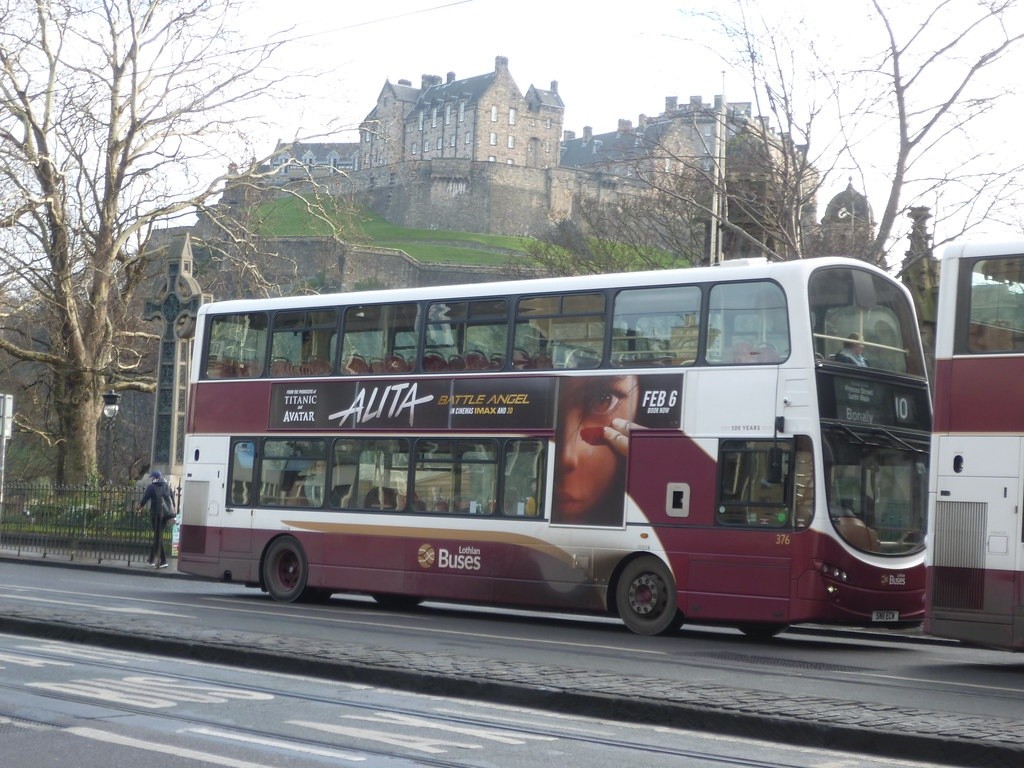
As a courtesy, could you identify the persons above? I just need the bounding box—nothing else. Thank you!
[552,374,650,525]
[134,470,177,569]
[834,332,871,367]
[304,458,326,505]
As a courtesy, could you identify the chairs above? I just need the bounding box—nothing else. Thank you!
[756,342,778,364]
[365,488,395,511]
[345,354,369,375]
[384,352,407,374]
[207,354,236,377]
[270,357,292,378]
[407,356,416,373]
[446,355,465,372]
[490,353,505,370]
[424,350,447,372]
[291,360,308,375]
[308,355,332,375]
[463,349,488,372]
[733,340,756,364]
[532,351,554,369]
[987,320,1014,351]
[238,361,261,377]
[511,348,531,370]
[369,357,384,373]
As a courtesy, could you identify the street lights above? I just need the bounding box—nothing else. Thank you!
[101,389,123,489]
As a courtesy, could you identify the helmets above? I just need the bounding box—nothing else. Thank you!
[148,471,161,478]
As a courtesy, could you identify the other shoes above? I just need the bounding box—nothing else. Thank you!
[160,560,168,568]
[146,563,155,567]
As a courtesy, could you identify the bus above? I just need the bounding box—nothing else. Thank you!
[923,241,1023,651]
[176,255,933,639]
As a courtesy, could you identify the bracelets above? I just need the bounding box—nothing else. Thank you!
[136,507,142,511]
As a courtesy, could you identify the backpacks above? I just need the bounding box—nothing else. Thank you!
[154,485,176,518]
